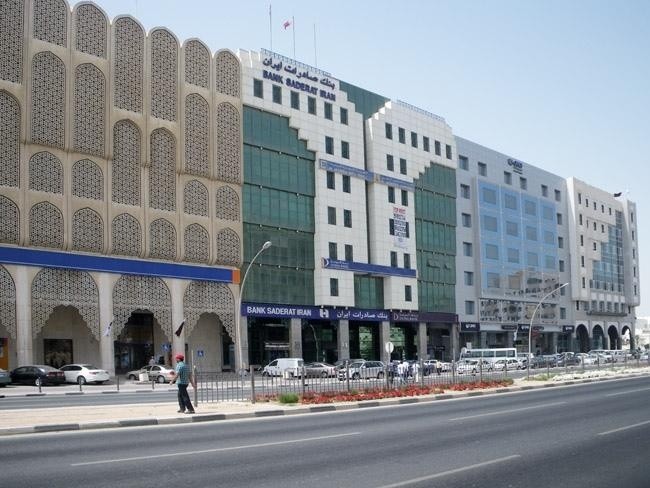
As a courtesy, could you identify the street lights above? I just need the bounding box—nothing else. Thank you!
[528,280,570,371]
[238,239,273,374]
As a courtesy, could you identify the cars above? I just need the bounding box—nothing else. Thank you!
[519,348,649,370]
[0,368,12,386]
[457,359,522,377]
[9,364,66,387]
[305,357,456,379]
[126,363,177,383]
[59,362,110,385]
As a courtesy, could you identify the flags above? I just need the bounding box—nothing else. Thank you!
[283,20,290,30]
[614,192,622,197]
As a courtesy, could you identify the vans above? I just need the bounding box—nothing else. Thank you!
[261,358,308,380]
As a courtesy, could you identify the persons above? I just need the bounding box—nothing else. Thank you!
[411,360,420,383]
[403,359,410,383]
[387,359,396,383]
[397,361,407,384]
[168,354,198,414]
[148,355,156,365]
[436,359,442,375]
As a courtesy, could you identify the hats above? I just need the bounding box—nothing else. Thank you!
[175,353,184,361]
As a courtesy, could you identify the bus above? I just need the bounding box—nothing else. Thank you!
[459,348,518,362]
